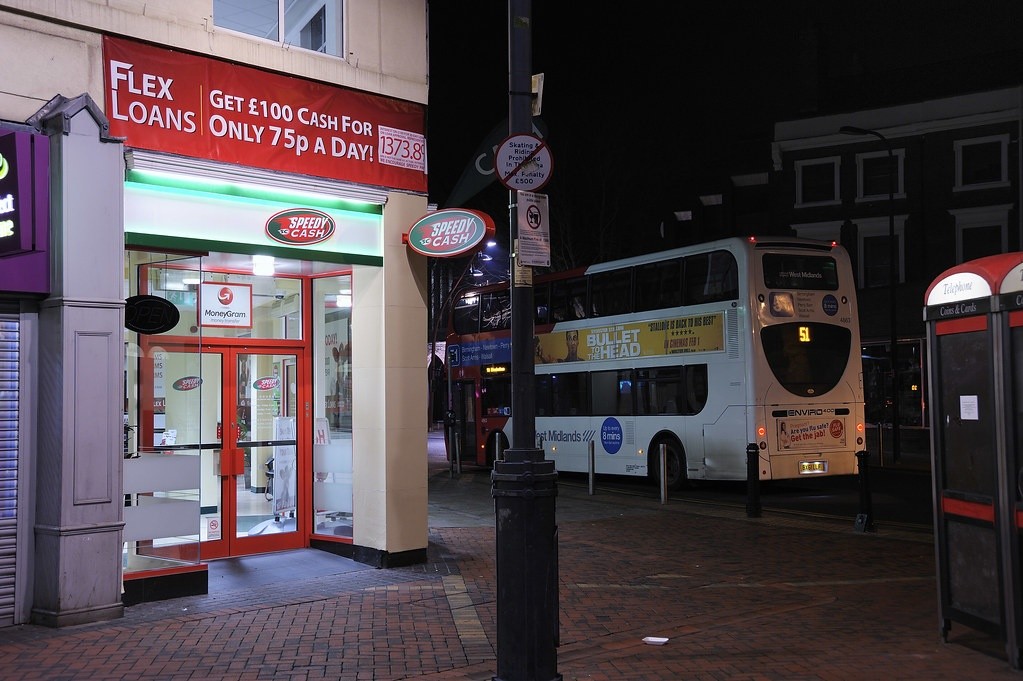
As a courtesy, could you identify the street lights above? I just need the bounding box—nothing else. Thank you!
[838,124,904,464]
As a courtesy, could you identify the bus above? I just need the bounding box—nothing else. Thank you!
[442,234,870,495]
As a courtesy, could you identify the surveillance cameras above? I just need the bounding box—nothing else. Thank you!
[274,290,287,300]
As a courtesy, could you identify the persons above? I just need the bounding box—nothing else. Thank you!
[564,330,582,361]
[573,296,597,317]
